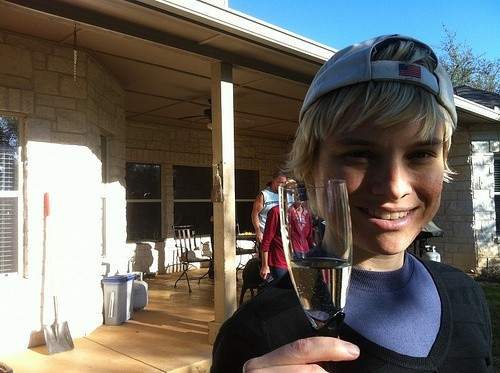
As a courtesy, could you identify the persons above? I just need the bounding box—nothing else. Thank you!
[259,179,319,283]
[210,33,494,373]
[251,169,295,245]
[208,202,238,282]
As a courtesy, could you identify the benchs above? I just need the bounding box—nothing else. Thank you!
[236,248,258,271]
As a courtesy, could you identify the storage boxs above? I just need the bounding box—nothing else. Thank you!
[101,274,136,326]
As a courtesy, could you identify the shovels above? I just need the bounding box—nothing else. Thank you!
[42,192,74,355]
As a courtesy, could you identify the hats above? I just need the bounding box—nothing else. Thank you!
[299,34,457,133]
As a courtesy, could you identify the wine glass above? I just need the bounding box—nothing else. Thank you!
[278,178,352,373]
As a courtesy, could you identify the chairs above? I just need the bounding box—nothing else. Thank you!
[239,258,262,305]
[171,225,211,293]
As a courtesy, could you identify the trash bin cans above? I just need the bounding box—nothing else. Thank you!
[101,275,136,325]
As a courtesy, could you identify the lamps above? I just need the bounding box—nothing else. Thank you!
[203,99,212,129]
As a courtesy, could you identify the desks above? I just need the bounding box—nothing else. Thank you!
[236,235,256,240]
[413,231,444,258]
